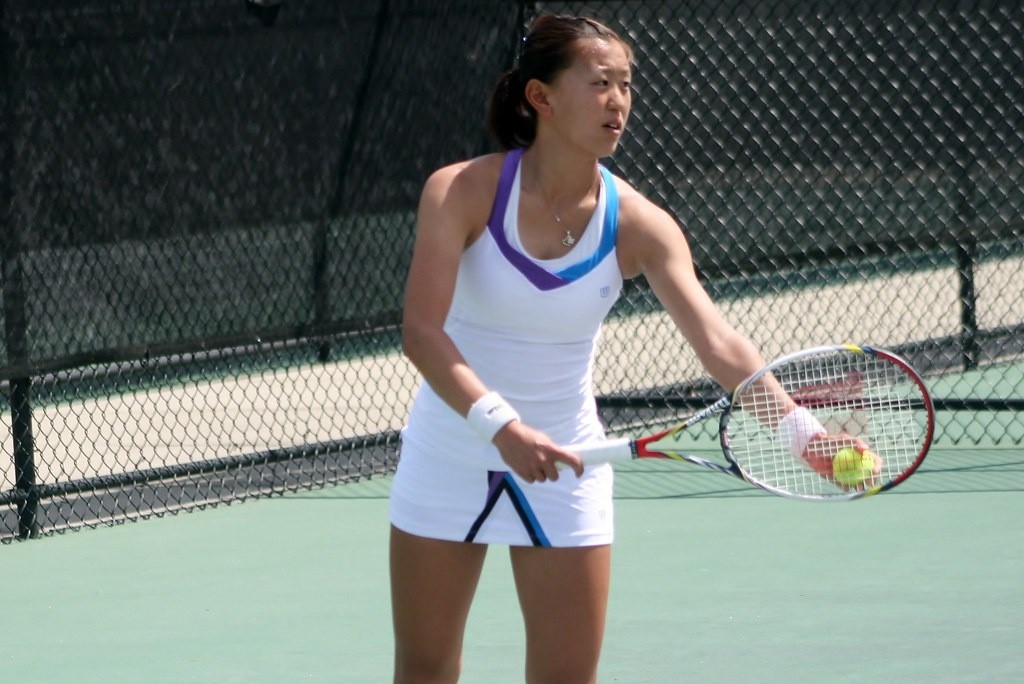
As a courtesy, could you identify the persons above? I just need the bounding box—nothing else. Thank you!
[389,14,881,684]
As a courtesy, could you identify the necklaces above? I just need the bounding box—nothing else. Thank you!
[527,162,598,246]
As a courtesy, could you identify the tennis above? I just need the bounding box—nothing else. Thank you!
[833,447,874,486]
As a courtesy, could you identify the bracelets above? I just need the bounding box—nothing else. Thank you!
[774,407,828,457]
[465,390,519,443]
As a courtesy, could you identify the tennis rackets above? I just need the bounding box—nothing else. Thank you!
[551,347,936,501]
